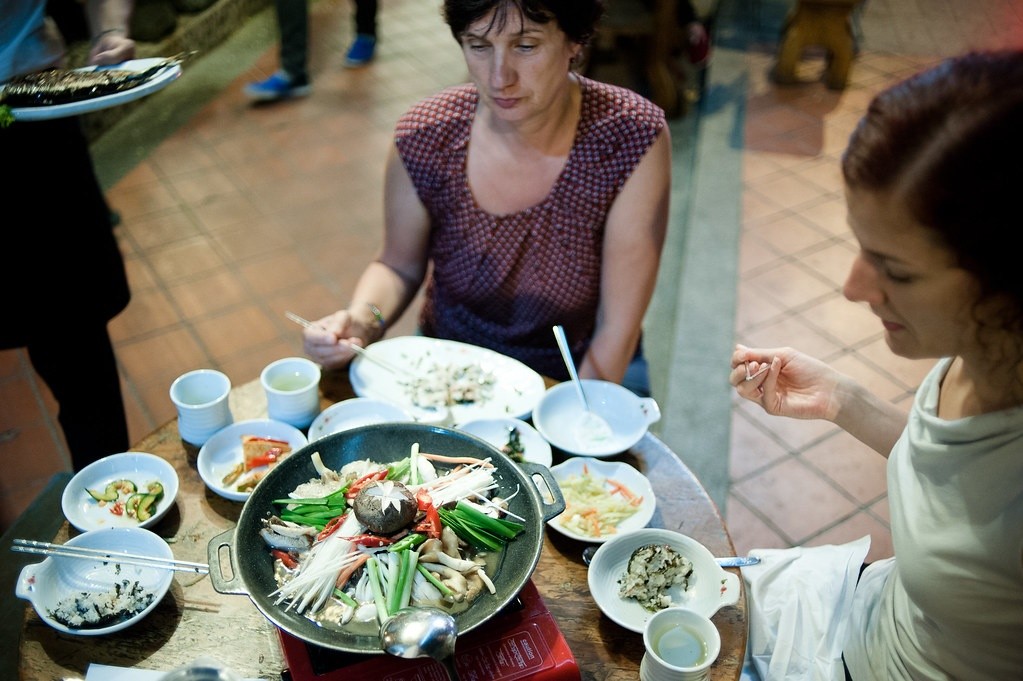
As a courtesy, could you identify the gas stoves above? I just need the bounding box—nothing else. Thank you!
[277,577,581,681]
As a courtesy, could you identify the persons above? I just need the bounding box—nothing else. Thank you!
[727,49,1023,681]
[299,0,671,410]
[0,0,137,473]
[244,0,381,97]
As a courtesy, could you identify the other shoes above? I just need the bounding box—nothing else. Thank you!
[740,6,786,42]
[343,34,377,63]
[126,0,175,43]
[242,69,311,100]
[710,20,747,50]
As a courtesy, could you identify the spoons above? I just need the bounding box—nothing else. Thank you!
[581,546,762,567]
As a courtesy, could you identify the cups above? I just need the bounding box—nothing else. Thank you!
[260,357,321,429]
[639,607,721,681]
[169,368,234,447]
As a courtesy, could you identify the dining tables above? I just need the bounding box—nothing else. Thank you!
[0,363,750,680]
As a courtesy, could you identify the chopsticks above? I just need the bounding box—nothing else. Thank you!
[283,311,396,373]
[744,360,773,382]
[10,538,210,574]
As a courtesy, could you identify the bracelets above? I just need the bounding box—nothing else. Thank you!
[91,27,124,45]
[348,301,385,337]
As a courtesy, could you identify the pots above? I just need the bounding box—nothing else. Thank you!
[207,421,567,654]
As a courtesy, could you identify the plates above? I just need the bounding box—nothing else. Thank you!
[197,418,308,502]
[62,452,179,529]
[349,334,547,421]
[307,397,416,444]
[0,57,183,121]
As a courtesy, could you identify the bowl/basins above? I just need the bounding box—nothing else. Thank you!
[16,527,174,635]
[454,416,553,484]
[588,528,740,635]
[537,457,656,543]
[531,379,662,457]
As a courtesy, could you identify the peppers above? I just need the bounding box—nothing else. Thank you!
[246,447,281,468]
[249,437,289,444]
[238,480,259,491]
[223,463,243,483]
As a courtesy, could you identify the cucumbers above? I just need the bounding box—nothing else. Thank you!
[84,480,163,521]
